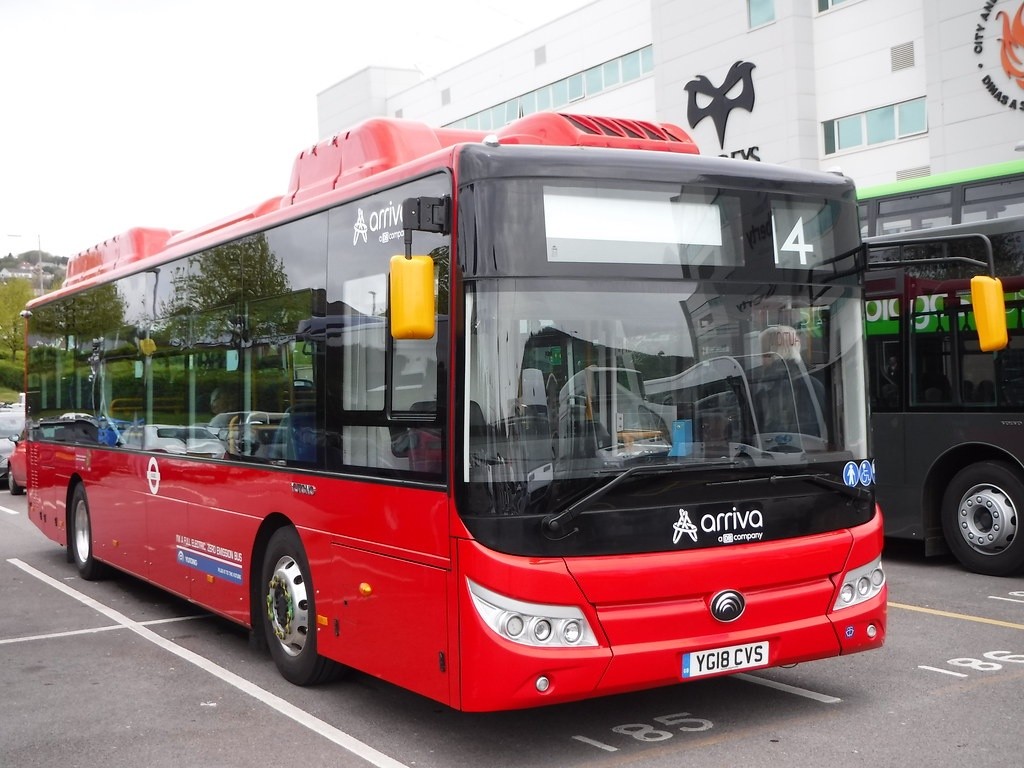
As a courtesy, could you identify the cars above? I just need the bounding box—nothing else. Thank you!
[96,417,145,446]
[0,402,30,494]
[121,424,231,461]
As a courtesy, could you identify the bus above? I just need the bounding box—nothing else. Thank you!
[855,160,1024,579]
[22,112,1009,713]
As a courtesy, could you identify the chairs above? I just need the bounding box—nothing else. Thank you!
[407,401,488,475]
[926,388,948,402]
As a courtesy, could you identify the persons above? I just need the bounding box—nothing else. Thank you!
[738,323,825,452]
[881,352,925,404]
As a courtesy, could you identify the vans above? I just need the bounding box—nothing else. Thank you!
[210,411,290,458]
